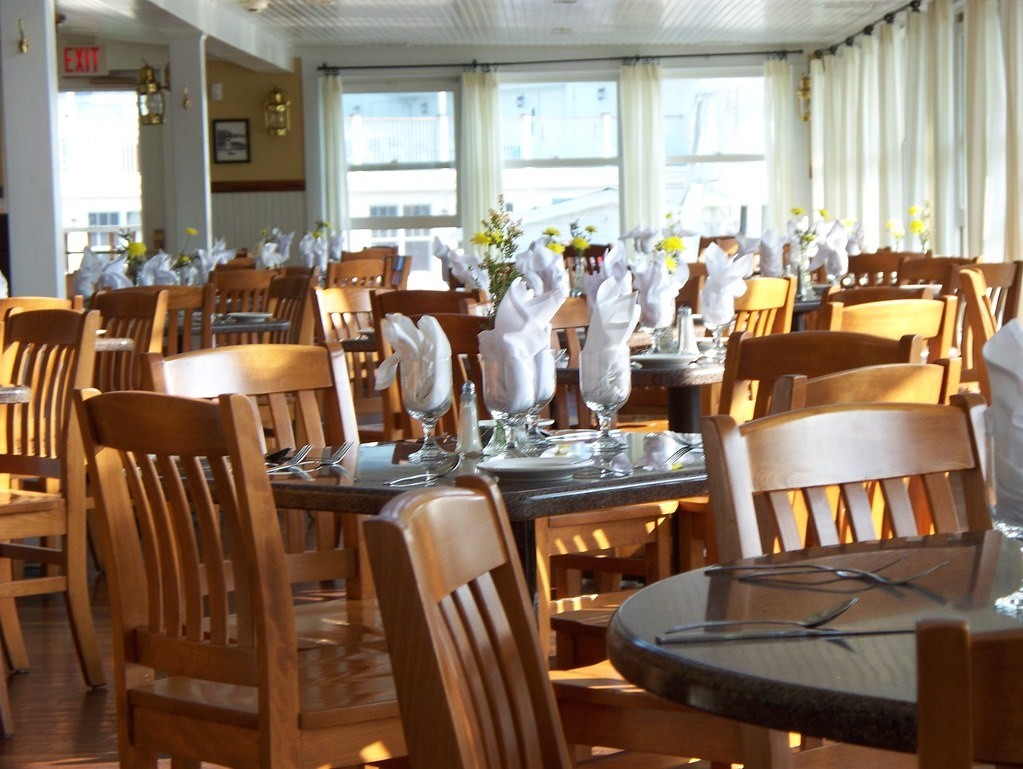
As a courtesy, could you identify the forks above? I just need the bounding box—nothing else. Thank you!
[262,440,353,480]
[701,562,954,584]
[670,433,703,446]
[736,555,906,580]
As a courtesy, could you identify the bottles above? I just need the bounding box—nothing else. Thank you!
[453,380,483,455]
[675,306,700,356]
[784,265,807,298]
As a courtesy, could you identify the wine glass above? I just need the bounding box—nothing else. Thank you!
[701,290,735,354]
[400,356,453,460]
[474,344,632,455]
[638,301,674,353]
[825,254,848,287]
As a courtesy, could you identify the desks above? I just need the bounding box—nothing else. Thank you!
[142,423,708,653]
[165,313,291,336]
[95,334,138,356]
[555,334,726,430]
[603,521,1023,769]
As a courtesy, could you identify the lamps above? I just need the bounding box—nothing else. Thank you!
[262,84,293,137]
[134,64,171,126]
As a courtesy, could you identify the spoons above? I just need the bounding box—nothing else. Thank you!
[664,596,859,632]
[382,453,461,485]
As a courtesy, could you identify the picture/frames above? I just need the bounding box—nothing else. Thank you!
[213,117,253,163]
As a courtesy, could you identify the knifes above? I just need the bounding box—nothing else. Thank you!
[654,624,916,646]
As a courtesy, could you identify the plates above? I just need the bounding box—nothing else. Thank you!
[228,312,273,324]
[476,457,594,481]
[478,417,555,429]
[629,352,698,368]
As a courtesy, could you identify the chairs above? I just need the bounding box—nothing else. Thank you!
[816,281,937,330]
[939,256,1023,382]
[310,279,404,441]
[164,278,225,358]
[620,256,709,429]
[362,244,400,255]
[716,326,930,419]
[894,255,984,356]
[206,269,273,344]
[782,242,828,282]
[549,355,965,769]
[916,615,1023,769]
[326,255,394,289]
[256,261,326,345]
[0,301,105,739]
[66,380,408,769]
[369,282,487,442]
[365,474,578,769]
[394,309,500,435]
[693,390,994,557]
[847,247,934,287]
[391,253,415,290]
[339,247,393,259]
[703,273,799,337]
[94,285,170,387]
[821,296,958,364]
[955,267,1003,402]
[542,294,591,431]
[144,341,417,654]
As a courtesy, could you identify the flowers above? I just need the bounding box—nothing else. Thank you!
[170,226,200,271]
[116,224,149,284]
[252,227,274,256]
[540,227,566,254]
[311,218,330,241]
[886,217,905,250]
[569,217,598,258]
[789,206,833,279]
[653,209,687,274]
[906,198,935,252]
[472,192,526,329]
